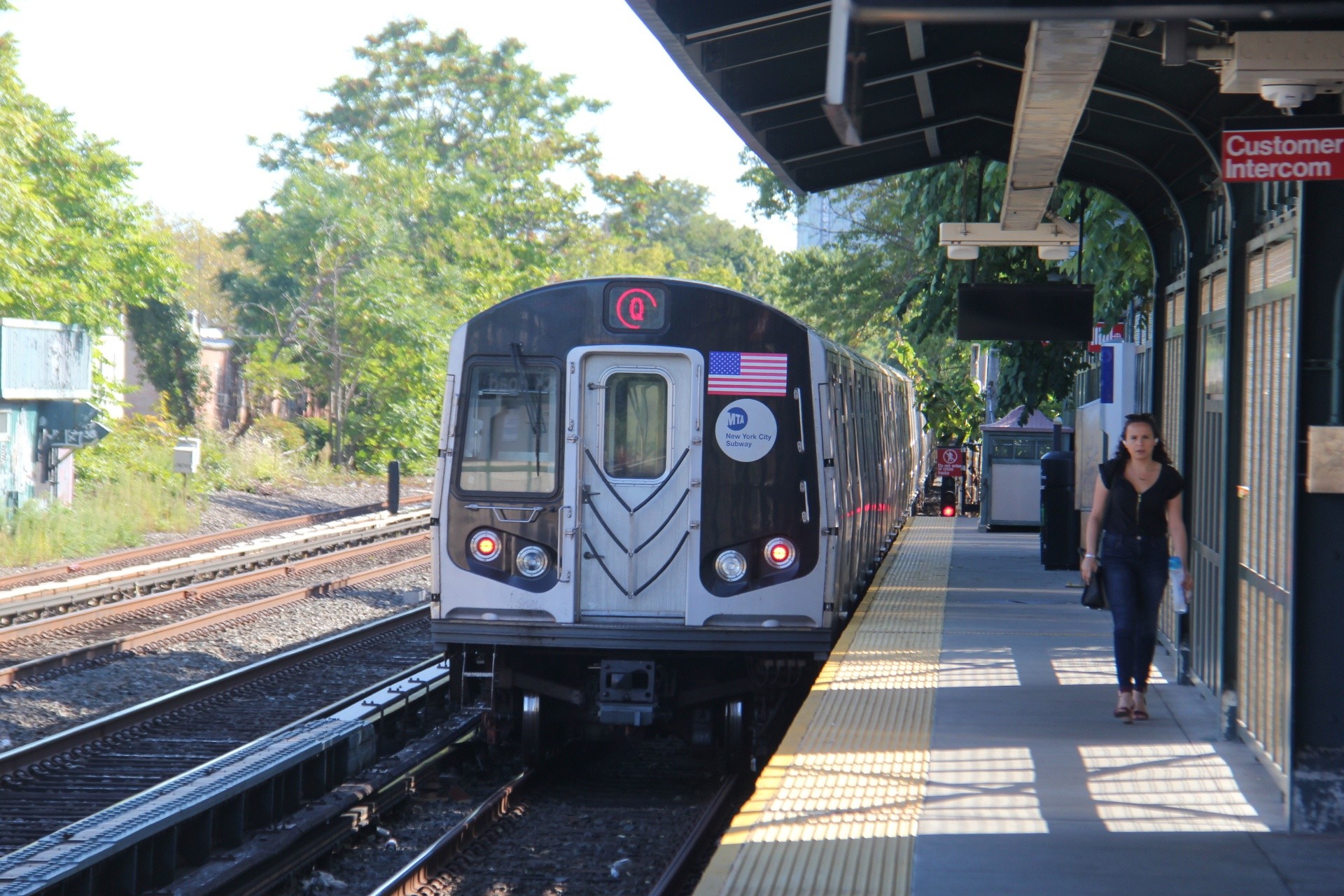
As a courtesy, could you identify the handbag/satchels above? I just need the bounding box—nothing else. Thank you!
[1076,547,1105,610]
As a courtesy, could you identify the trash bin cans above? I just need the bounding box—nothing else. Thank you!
[1038,450,1081,571]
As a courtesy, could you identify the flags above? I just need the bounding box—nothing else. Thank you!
[707,351,788,397]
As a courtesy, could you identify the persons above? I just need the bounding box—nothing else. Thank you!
[1081,412,1194,724]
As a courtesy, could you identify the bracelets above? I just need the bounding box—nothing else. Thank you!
[1084,555,1100,561]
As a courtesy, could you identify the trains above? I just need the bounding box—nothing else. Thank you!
[425,274,939,770]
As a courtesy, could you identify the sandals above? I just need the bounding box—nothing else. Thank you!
[1113,702,1135,717]
[1132,697,1149,721]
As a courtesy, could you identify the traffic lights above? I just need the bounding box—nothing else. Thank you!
[941,476,957,516]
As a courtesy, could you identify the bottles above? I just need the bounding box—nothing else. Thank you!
[1169,556,1187,615]
